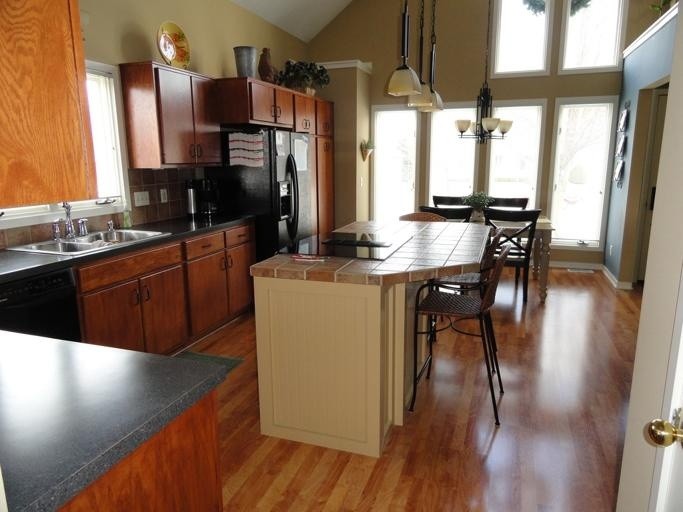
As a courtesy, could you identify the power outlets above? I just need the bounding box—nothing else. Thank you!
[161,189,167,204]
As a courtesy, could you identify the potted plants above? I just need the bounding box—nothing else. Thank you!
[276,59,330,96]
[463,191,495,221]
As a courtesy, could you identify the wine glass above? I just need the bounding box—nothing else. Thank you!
[202,178,216,215]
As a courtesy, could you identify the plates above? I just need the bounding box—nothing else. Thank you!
[157,20,191,70]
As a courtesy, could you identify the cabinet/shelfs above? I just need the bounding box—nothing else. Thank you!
[118,60,223,170]
[316,136,337,257]
[74,242,189,356]
[183,223,256,349]
[253,278,431,460]
[315,98,335,137]
[215,78,295,129]
[294,91,316,132]
[0,0,101,208]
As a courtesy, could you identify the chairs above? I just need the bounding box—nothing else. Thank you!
[407,246,513,425]
[398,213,447,222]
[429,227,503,352]
[489,196,529,208]
[433,195,470,204]
[419,206,473,221]
[484,207,542,303]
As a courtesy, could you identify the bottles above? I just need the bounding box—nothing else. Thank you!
[107,220,119,242]
[186,178,198,218]
[258,47,272,81]
[122,202,132,228]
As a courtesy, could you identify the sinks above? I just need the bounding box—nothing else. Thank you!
[4,240,112,255]
[73,228,174,247]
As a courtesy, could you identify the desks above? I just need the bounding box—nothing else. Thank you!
[469,217,557,304]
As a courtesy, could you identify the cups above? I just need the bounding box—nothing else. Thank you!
[233,45,257,77]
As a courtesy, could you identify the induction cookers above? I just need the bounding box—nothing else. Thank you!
[277,231,413,260]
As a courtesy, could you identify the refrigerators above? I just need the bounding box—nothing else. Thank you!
[222,129,313,262]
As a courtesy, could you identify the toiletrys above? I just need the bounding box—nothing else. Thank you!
[123,200,133,229]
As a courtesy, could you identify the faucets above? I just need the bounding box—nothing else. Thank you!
[61,200,76,239]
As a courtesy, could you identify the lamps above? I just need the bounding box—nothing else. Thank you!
[455,1,511,146]
[386,0,422,96]
[407,0,433,108]
[418,0,443,112]
